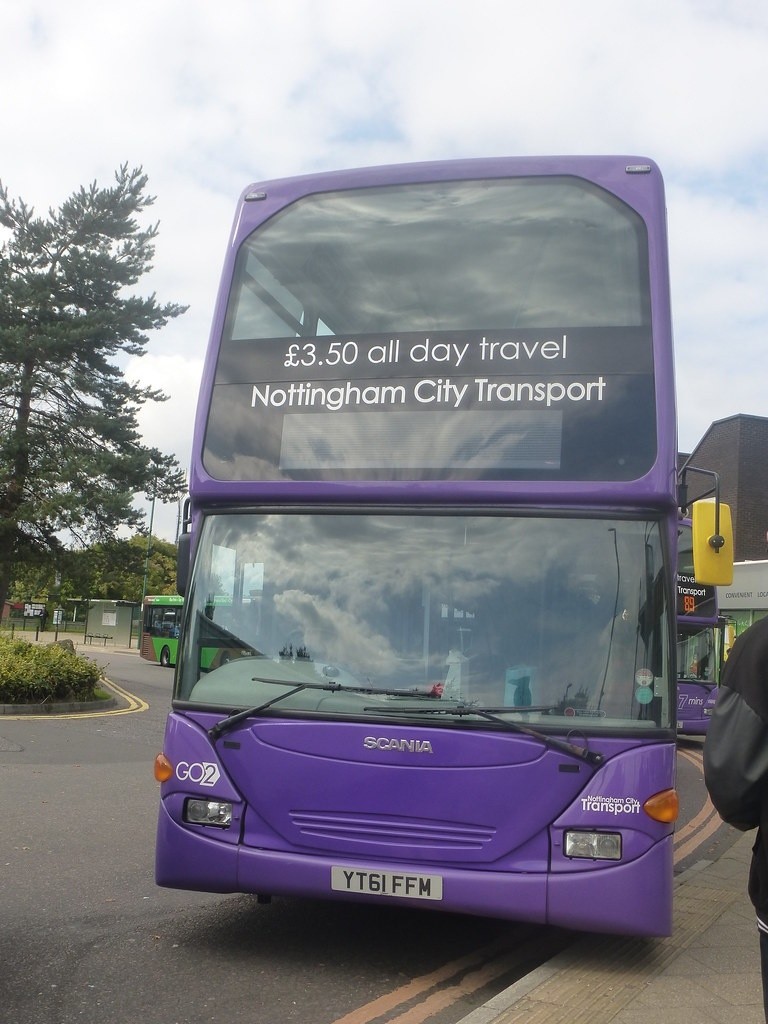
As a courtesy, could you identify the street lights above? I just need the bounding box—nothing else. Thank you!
[563,684,572,713]
[596,526,620,710]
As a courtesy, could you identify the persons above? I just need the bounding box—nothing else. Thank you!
[703,618,768,1024]
[152,613,161,627]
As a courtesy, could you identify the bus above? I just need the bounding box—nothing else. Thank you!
[676,517,735,737]
[153,154,737,940]
[140,594,262,669]
[676,572,735,734]
